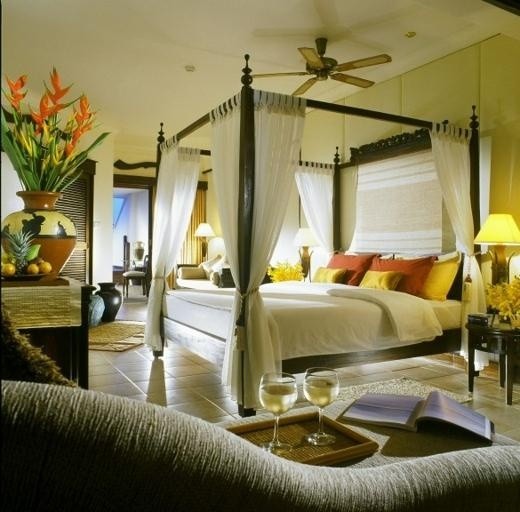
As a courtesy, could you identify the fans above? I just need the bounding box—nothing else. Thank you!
[252,38,392,96]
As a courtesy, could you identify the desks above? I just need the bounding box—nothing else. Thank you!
[465,316,520,405]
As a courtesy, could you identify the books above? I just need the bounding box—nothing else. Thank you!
[337,390,497,446]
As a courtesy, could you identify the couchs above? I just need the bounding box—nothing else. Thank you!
[2,381,519,511]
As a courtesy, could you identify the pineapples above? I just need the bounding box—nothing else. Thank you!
[4,225,36,274]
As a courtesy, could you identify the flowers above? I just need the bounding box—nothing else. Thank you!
[484,276,520,329]
[1,65,111,192]
[266,262,305,282]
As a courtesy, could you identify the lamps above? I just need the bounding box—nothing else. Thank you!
[292,228,322,284]
[473,213,520,284]
[194,223,216,263]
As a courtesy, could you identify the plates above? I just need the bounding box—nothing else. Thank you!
[1,274,46,280]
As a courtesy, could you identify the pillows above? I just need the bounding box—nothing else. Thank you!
[313,250,462,302]
[177,257,239,287]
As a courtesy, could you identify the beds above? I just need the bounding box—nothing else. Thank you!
[143,56,490,418]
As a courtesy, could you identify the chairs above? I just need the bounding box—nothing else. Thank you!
[112,236,130,297]
[122,255,150,298]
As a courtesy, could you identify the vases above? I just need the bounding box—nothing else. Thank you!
[134,241,144,261]
[499,315,512,329]
[88,282,122,327]
[1,193,78,276]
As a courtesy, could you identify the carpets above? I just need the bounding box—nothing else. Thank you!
[88,321,145,352]
[220,376,473,421]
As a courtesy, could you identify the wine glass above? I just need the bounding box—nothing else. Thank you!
[260,372,297,456]
[303,367,339,445]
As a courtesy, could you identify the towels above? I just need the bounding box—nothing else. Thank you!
[327,287,444,345]
